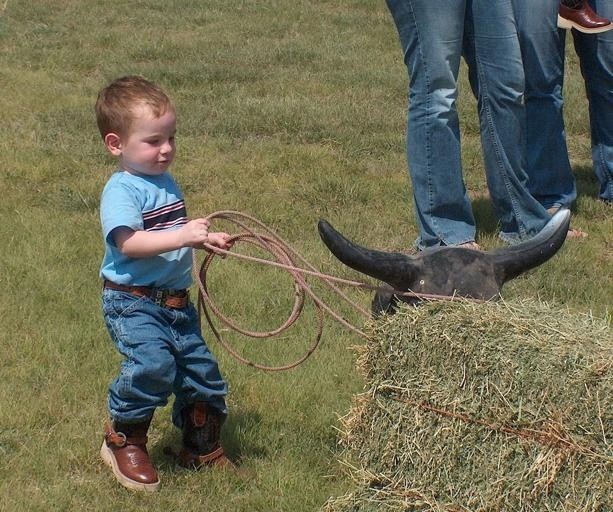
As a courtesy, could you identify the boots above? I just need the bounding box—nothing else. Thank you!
[100,420,161,493]
[180,401,238,474]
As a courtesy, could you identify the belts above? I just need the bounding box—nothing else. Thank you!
[104,281,190,308]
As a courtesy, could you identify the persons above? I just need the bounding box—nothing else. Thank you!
[385,0,588,251]
[511,0,613,215]
[95,76,235,492]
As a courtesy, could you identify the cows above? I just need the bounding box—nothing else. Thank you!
[316,203,574,320]
